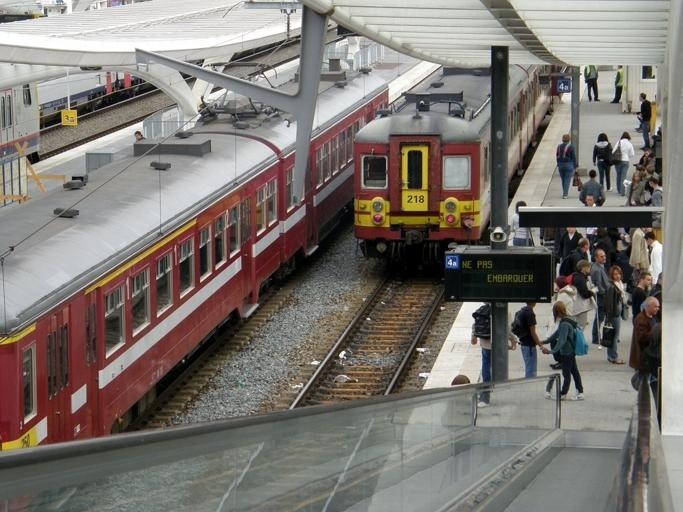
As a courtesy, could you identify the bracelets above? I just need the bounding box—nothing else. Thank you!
[539,346,544,352]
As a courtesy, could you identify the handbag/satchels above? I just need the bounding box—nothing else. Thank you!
[573,172,579,186]
[609,149,621,165]
[602,322,615,347]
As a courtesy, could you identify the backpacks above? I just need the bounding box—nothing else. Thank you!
[472,304,491,339]
[568,327,588,355]
[512,309,529,337]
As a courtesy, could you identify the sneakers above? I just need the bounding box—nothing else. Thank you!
[477,402,490,408]
[545,392,584,400]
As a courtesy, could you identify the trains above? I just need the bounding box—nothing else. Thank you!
[353,64,562,280]
[0,52,390,459]
[37,69,188,119]
[0,83,40,161]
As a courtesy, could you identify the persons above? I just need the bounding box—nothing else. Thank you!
[535,128,661,404]
[582,65,601,103]
[636,92,651,151]
[609,65,625,103]
[510,200,533,247]
[511,301,550,378]
[135,131,146,142]
[468,299,517,406]
[442,375,472,485]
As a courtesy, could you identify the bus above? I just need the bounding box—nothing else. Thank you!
[0,13,47,22]
